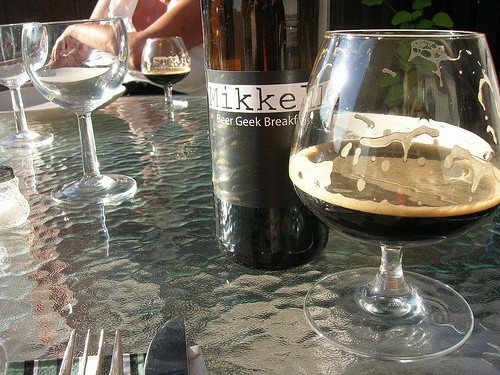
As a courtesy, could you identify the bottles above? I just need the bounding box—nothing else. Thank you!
[201,0,329,272]
[0,167,31,231]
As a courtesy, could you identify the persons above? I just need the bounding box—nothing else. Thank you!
[36,0,209,99]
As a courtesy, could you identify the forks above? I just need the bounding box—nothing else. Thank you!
[57,329,125,375]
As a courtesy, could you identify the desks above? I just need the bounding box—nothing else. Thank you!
[1,92,499,374]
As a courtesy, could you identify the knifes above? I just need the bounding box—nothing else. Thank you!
[143,317,189,375]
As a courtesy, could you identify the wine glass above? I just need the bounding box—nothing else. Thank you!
[153,110,195,162]
[140,36,192,113]
[22,16,139,208]
[288,30,500,362]
[0,22,55,151]
